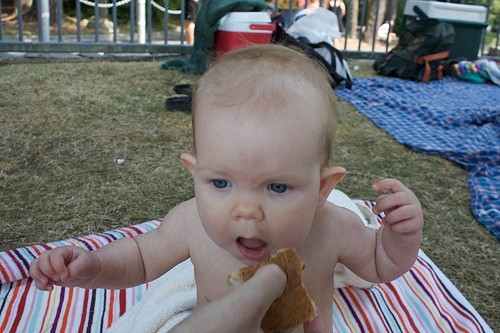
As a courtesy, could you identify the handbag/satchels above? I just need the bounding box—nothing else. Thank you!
[270,24,353,89]
[286,6,351,78]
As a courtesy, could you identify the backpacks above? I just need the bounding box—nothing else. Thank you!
[373,5,456,82]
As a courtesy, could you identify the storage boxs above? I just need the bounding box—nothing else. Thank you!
[213,11,277,57]
[403,1,488,62]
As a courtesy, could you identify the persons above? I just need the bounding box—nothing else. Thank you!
[182,0,394,47]
[30,43,424,333]
[165,264,305,333]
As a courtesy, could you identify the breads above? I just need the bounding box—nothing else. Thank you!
[236,246,318,333]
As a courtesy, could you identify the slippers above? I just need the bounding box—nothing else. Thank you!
[166,96,192,111]
[174,83,194,94]
